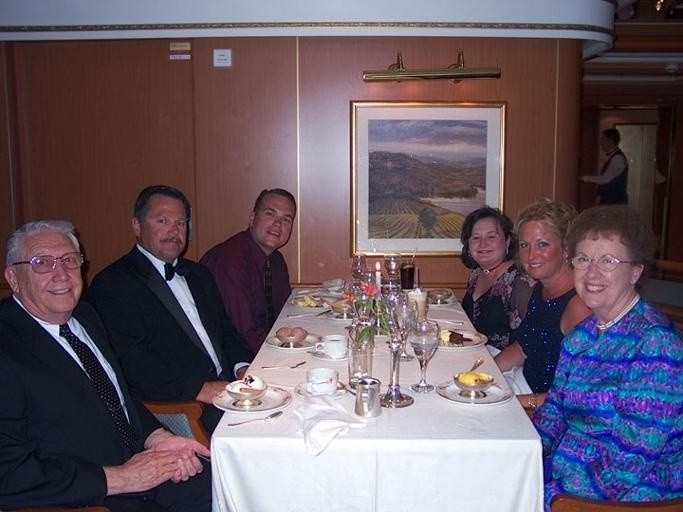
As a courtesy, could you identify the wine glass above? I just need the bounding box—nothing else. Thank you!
[379,250,438,407]
[454,371,494,398]
[277,330,307,347]
[429,288,453,304]
[227,385,269,407]
[330,303,353,318]
[350,253,375,337]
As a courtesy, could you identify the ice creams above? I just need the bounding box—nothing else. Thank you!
[458,371,492,385]
[226,374,265,393]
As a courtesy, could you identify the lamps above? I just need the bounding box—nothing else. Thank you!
[361,49,502,84]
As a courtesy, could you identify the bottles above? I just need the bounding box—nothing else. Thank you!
[354,378,381,419]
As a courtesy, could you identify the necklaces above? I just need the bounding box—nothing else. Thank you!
[480,261,507,274]
[597,293,641,331]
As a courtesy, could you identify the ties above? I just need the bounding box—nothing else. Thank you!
[59,323,137,454]
[263,255,276,330]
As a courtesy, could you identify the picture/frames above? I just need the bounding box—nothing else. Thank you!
[350,101,505,260]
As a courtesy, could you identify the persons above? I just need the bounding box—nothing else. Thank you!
[530,205,683,512]
[85,184,256,437]
[0,219,211,512]
[199,188,297,355]
[577,127,629,206]
[460,206,537,357]
[493,198,592,417]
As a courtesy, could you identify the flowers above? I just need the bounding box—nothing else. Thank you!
[356,278,396,344]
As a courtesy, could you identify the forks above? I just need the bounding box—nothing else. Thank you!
[287,308,328,321]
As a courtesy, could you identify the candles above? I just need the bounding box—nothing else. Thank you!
[374,261,382,295]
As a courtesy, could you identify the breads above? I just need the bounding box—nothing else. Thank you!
[291,328,307,336]
[298,296,315,307]
[275,327,291,336]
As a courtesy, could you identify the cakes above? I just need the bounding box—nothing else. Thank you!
[439,329,462,345]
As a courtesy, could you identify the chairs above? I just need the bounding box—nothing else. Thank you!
[547,493,682,512]
[0,399,211,512]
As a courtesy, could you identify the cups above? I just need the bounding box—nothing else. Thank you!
[316,335,347,358]
[306,367,339,394]
[348,344,371,388]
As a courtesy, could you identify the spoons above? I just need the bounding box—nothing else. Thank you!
[227,411,284,429]
[256,360,307,371]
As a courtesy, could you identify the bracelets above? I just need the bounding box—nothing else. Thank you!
[529,394,538,412]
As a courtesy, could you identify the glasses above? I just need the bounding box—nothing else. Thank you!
[568,253,642,270]
[9,251,85,274]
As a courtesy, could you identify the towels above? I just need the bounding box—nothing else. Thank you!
[291,392,368,460]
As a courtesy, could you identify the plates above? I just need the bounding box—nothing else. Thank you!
[211,386,291,412]
[288,285,350,308]
[325,310,351,322]
[295,382,349,400]
[437,379,514,406]
[267,334,320,352]
[427,297,460,306]
[311,348,352,360]
[436,329,489,349]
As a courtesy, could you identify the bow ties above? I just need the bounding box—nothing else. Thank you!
[163,261,187,281]
[605,146,619,155]
